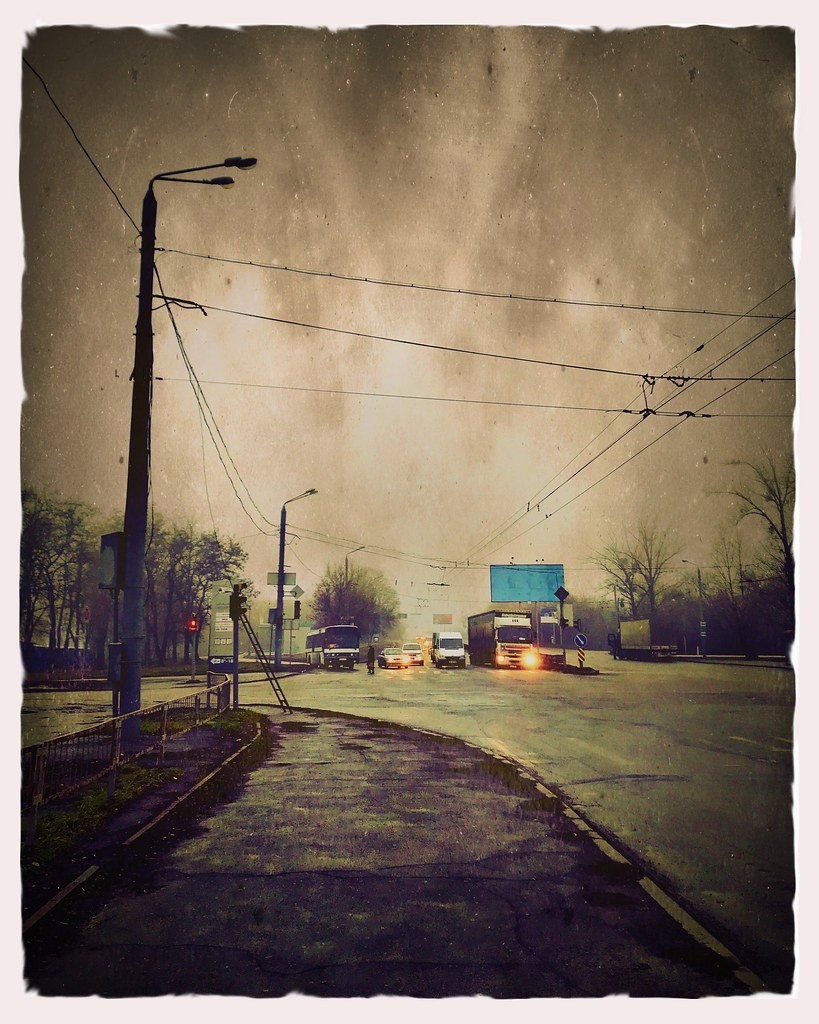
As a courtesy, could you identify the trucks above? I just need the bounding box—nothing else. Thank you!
[468,609,533,668]
[403,643,424,666]
[608,618,678,660]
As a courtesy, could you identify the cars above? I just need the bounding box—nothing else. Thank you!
[378,648,411,668]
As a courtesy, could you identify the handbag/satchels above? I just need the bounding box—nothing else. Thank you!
[367,663,374,670]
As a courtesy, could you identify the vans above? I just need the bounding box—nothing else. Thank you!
[431,632,465,668]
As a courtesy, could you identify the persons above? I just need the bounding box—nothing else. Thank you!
[366,645,375,674]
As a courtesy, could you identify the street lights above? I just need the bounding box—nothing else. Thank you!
[274,488,320,666]
[682,560,708,655]
[120,157,260,738]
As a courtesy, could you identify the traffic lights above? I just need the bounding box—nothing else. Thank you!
[561,617,569,630]
[189,618,199,631]
[573,619,582,632]
[230,593,249,620]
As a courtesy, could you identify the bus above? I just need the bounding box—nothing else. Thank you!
[305,625,360,669]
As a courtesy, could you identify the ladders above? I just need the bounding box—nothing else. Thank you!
[240,613,292,714]
